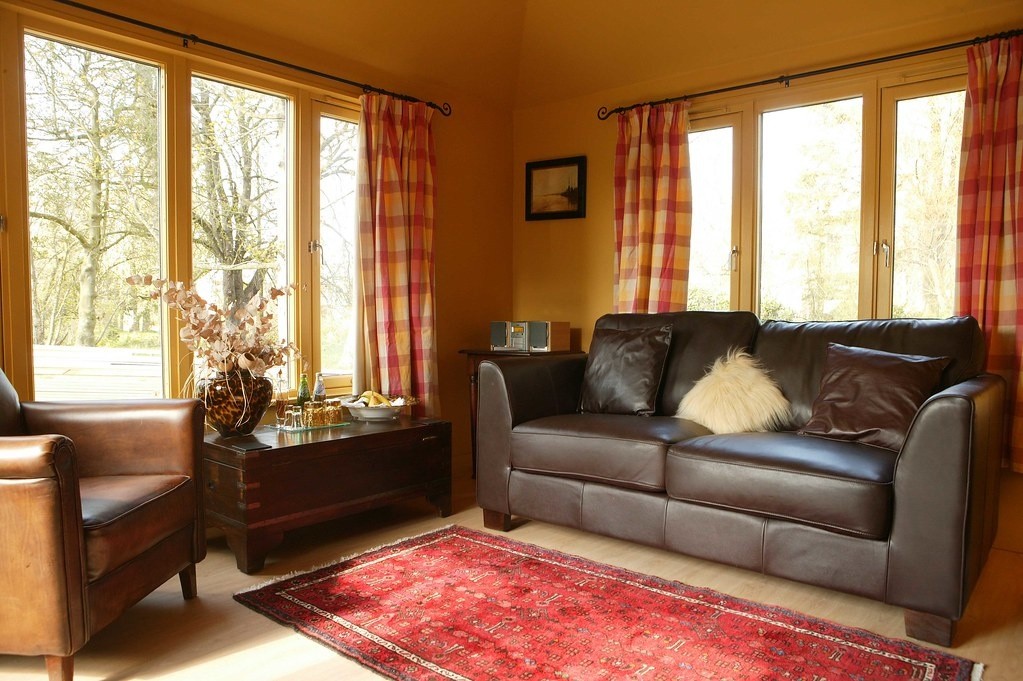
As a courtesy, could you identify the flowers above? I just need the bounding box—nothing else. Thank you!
[120,259,313,438]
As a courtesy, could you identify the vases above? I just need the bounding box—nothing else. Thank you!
[195,369,273,438]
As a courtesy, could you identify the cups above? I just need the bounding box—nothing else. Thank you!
[285,399,341,428]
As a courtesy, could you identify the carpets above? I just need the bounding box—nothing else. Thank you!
[230,523,984,681]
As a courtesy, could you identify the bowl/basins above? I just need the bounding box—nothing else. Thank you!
[340,405,406,422]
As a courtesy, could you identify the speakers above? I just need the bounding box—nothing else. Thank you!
[530,320,569,352]
[488,321,510,350]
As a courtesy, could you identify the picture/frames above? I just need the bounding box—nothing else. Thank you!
[524,152,587,221]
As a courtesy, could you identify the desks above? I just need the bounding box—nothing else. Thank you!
[204,412,453,574]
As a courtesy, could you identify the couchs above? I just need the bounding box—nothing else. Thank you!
[0,366,206,681]
[475,312,1005,649]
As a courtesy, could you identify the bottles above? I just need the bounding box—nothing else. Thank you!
[275,369,288,420]
[297,373,310,408]
[313,372,325,401]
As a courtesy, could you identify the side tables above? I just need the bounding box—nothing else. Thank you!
[459,349,585,482]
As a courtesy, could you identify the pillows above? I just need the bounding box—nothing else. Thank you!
[576,321,674,417]
[797,343,952,453]
